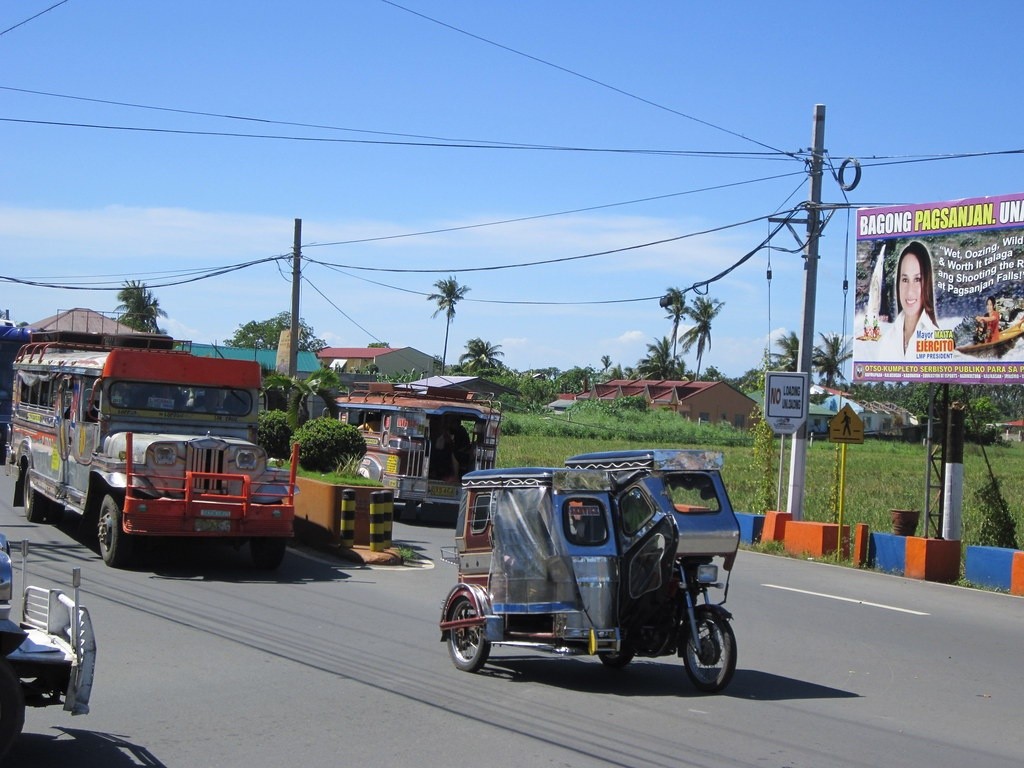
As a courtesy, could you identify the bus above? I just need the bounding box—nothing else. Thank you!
[3,331,300,574]
[334,386,506,527]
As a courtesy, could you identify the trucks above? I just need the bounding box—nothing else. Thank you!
[0,538,97,764]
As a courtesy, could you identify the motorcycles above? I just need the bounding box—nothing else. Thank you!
[438,449,737,695]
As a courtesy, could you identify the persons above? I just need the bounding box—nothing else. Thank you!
[879,241,955,359]
[863,314,880,337]
[974,297,1002,341]
[432,412,469,481]
